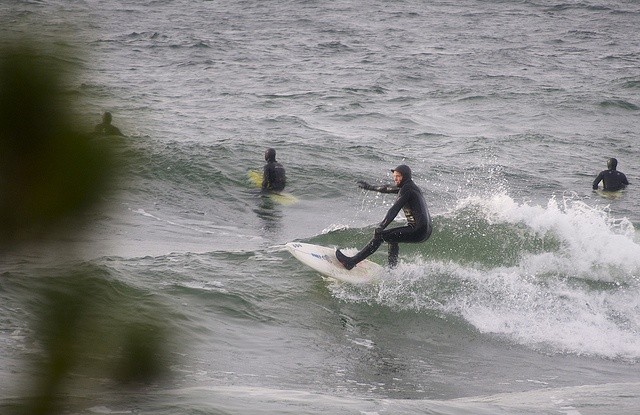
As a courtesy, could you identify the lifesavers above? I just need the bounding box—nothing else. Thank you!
[595,179,624,199]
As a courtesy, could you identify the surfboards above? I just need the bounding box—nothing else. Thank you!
[286,242,384,285]
[247,169,297,207]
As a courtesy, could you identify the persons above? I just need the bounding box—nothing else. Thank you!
[592,159,628,192]
[94,112,122,135]
[259,149,286,192]
[335,164,433,271]
